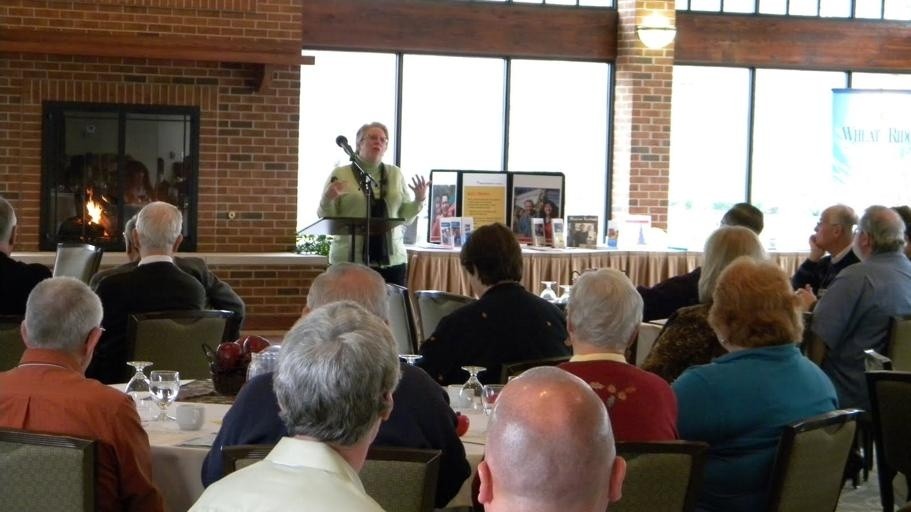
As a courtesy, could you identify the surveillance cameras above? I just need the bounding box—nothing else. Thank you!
[83,118,96,136]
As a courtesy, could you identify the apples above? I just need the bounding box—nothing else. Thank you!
[216,335,272,369]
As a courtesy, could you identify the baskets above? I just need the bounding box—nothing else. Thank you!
[200,334,273,396]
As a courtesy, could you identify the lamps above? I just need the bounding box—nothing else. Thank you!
[635,12,678,50]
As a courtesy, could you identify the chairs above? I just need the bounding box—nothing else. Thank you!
[0,243,909,512]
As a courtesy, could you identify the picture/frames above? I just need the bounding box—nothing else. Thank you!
[507,170,564,246]
[426,167,460,244]
[457,170,510,231]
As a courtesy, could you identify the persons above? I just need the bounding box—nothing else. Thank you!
[202,262,472,508]
[790,204,911,411]
[0,276,164,512]
[478,366,626,512]
[637,202,767,382]
[558,267,678,443]
[88,201,245,384]
[315,121,435,282]
[412,225,573,390]
[431,193,455,239]
[518,201,557,237]
[670,256,839,512]
[187,300,402,512]
[0,201,52,319]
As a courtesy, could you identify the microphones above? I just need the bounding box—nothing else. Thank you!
[336,136,362,164]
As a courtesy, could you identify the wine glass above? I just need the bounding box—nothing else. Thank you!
[457,365,518,419]
[124,359,180,423]
[540,280,572,303]
[399,354,423,365]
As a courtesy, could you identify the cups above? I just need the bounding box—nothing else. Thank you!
[176,404,206,431]
[247,352,278,384]
